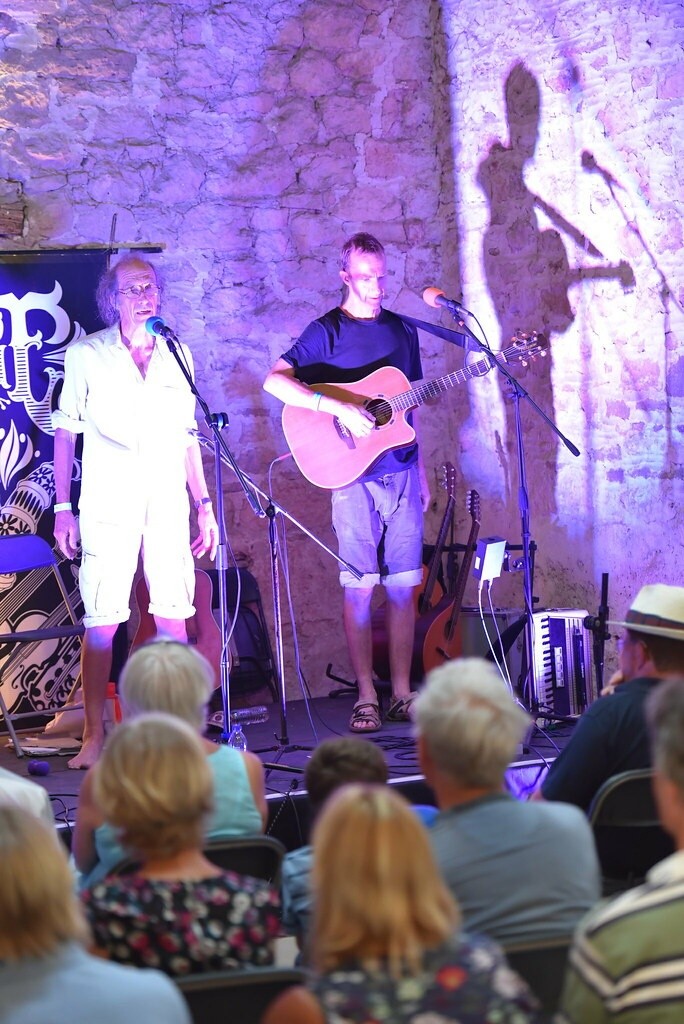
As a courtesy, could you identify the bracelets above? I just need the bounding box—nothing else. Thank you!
[194,498,211,509]
[54,503,72,513]
[310,391,323,412]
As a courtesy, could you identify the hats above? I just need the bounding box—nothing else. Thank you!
[603,583,684,640]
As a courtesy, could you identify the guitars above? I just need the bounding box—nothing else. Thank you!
[280,327,547,490]
[370,462,457,682]
[120,567,232,691]
[410,489,482,692]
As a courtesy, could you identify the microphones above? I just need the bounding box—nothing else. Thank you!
[185,422,206,440]
[423,287,473,317]
[146,316,178,341]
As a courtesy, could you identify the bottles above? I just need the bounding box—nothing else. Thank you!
[230,705,270,726]
[228,726,247,752]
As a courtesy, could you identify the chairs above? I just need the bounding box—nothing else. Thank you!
[205,567,279,702]
[0,534,86,757]
[173,768,678,1024]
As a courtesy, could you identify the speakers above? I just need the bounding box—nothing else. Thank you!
[456,606,530,688]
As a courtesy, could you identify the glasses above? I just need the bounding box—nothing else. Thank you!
[114,284,161,299]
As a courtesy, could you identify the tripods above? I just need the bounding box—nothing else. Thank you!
[448,310,581,753]
[195,437,365,782]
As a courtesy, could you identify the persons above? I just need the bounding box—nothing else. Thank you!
[264,782,543,1024]
[77,714,282,976]
[0,790,190,1024]
[552,676,684,1024]
[281,736,389,944]
[51,257,220,769]
[411,655,601,947]
[263,232,431,731]
[72,637,268,885]
[531,583,684,806]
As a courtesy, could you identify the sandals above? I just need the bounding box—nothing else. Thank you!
[349,698,382,733]
[383,692,420,720]
